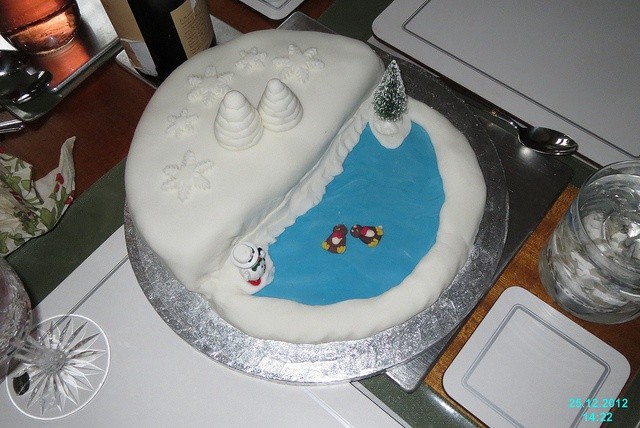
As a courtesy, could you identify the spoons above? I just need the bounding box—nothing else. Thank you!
[434,76,579,157]
[0,68,55,106]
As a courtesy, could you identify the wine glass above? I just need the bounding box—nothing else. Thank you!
[0,256,111,419]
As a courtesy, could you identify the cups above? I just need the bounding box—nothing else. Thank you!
[540,160,640,325]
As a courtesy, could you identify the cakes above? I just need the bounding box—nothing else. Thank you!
[124,29,487,346]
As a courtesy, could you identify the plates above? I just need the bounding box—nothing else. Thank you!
[371,0,640,176]
[441,284,632,428]
[123,49,511,384]
[239,0,307,21]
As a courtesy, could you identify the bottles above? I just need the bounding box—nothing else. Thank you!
[100,0,217,87]
[0,0,81,56]
